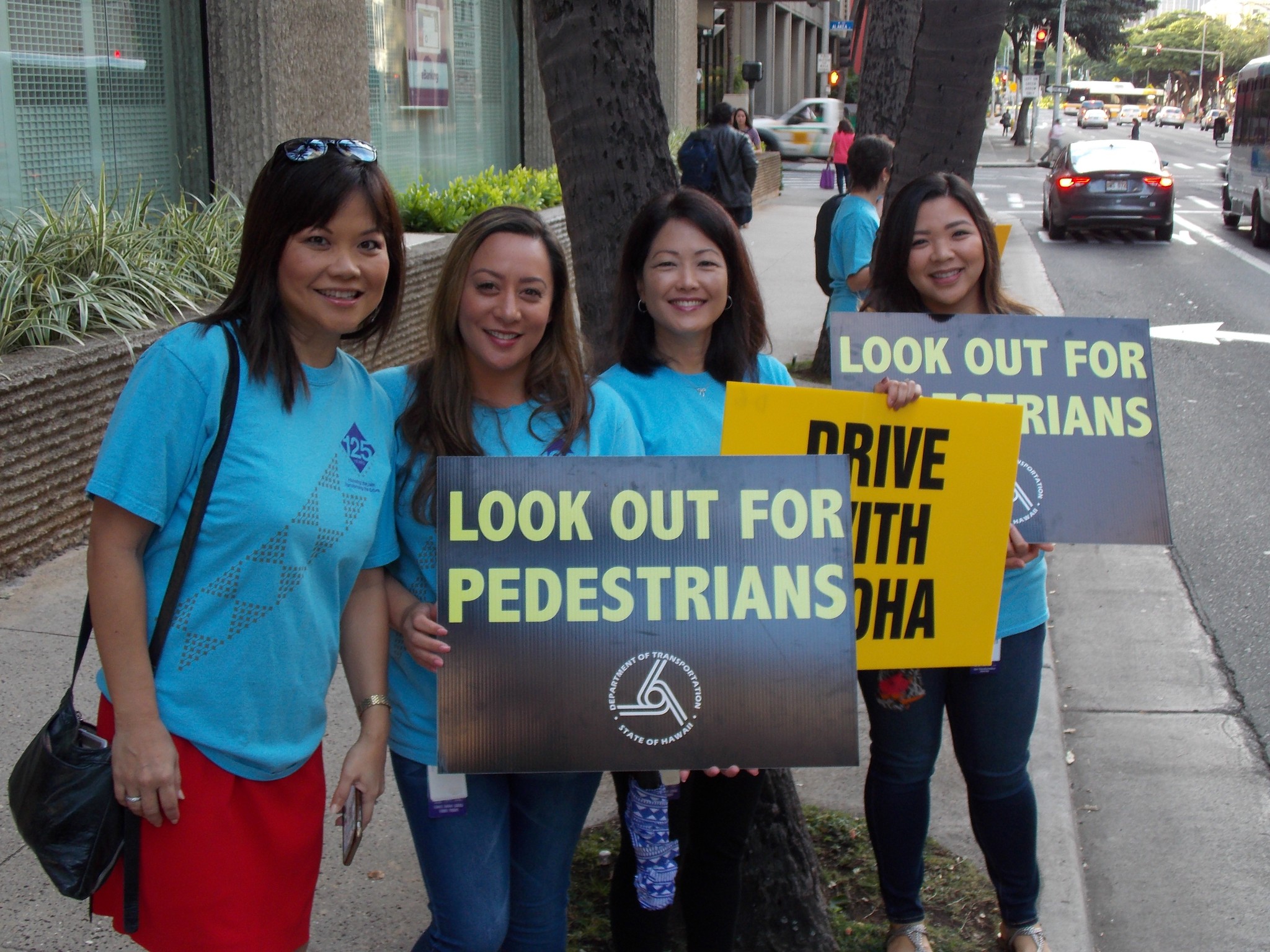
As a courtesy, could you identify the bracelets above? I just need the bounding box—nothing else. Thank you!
[356,695,390,720]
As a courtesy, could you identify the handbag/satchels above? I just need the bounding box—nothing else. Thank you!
[1000,117,1004,124]
[819,163,835,188]
[7,695,141,902]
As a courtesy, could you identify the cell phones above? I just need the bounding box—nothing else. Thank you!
[342,785,363,866]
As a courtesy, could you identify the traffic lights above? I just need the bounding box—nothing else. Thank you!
[827,71,842,86]
[713,8,726,39]
[1002,74,1007,92]
[1034,28,1047,68]
[839,37,851,67]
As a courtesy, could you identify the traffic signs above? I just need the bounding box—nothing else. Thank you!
[1044,85,1071,94]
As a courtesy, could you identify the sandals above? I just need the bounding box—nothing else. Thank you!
[885,923,933,952]
[996,919,1048,952]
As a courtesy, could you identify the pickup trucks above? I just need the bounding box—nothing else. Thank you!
[753,97,857,162]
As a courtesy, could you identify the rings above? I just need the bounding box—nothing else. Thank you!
[126,796,141,802]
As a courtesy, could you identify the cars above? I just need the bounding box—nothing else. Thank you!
[1116,104,1142,126]
[1081,109,1109,129]
[1038,139,1175,242]
[1155,106,1185,130]
[1201,109,1230,133]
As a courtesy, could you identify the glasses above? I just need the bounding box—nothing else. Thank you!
[283,138,378,164]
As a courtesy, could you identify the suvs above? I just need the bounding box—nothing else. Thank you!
[1076,100,1105,127]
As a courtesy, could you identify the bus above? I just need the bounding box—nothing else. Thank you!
[1213,54,1270,249]
[1064,80,1164,122]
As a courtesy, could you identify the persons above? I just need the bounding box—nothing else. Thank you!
[877,192,884,220]
[597,190,922,952]
[734,101,1206,192]
[856,173,1054,952]
[369,206,603,952]
[825,134,894,351]
[83,137,401,952]
[679,102,757,229]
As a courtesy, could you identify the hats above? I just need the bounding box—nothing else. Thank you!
[714,102,735,119]
